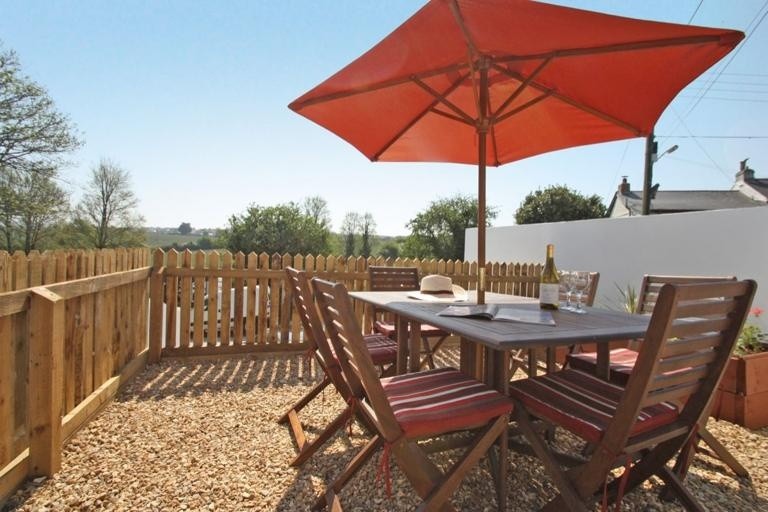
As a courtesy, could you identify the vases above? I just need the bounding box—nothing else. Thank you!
[707,346,766,433]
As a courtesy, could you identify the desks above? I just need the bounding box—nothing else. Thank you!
[343,286,687,510]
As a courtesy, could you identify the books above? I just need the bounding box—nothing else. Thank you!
[436,304,556,326]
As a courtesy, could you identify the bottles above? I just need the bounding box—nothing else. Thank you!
[538,244,561,308]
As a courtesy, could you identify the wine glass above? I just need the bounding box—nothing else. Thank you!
[560,271,593,315]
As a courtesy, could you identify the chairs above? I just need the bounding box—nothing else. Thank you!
[502,274,761,511]
[310,269,517,510]
[275,260,416,468]
[503,269,601,382]
[553,270,756,502]
[363,263,453,372]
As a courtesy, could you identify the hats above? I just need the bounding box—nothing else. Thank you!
[408,274,468,302]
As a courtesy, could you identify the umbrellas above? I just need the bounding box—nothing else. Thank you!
[287,0,746,304]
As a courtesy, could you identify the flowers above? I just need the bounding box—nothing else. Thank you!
[730,302,767,357]
